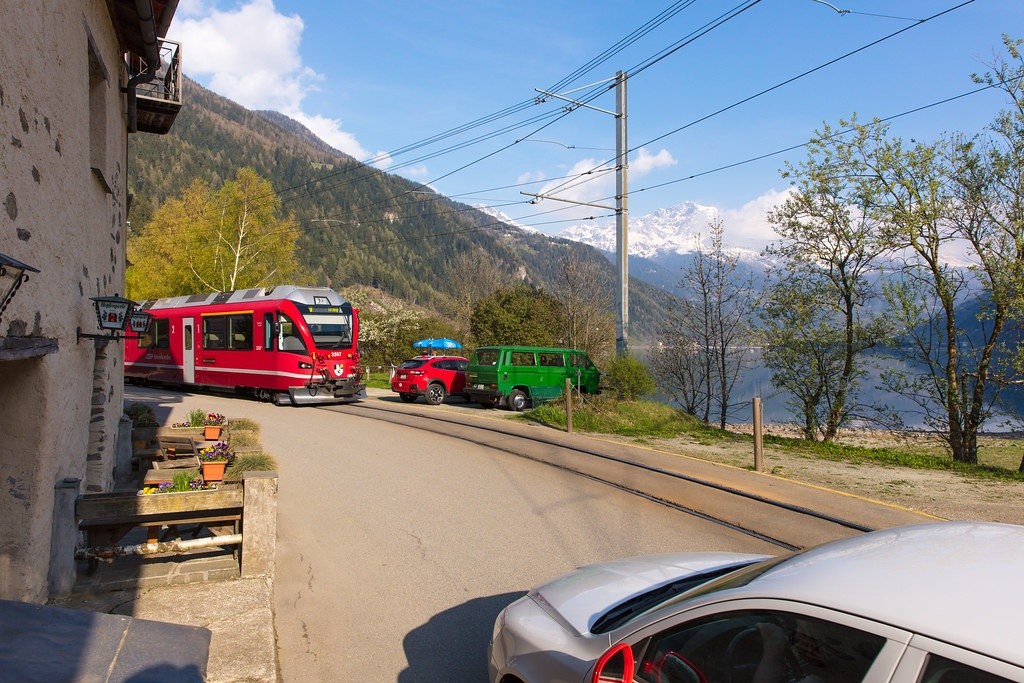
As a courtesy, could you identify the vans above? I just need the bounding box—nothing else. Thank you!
[466,346,602,411]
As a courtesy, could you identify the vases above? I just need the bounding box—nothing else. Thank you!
[170,424,205,435]
[204,426,222,440]
[201,461,227,481]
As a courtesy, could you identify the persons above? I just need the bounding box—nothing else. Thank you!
[752,619,859,683]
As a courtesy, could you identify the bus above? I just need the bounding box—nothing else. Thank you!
[123,286,368,407]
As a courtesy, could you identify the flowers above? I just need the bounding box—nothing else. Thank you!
[197,441,229,462]
[200,412,226,426]
[172,409,207,427]
[136,471,221,494]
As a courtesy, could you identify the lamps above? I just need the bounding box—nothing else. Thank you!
[116,310,156,341]
[74,292,142,346]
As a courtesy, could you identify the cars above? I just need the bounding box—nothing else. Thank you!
[485,516,1024,683]
[391,352,470,405]
